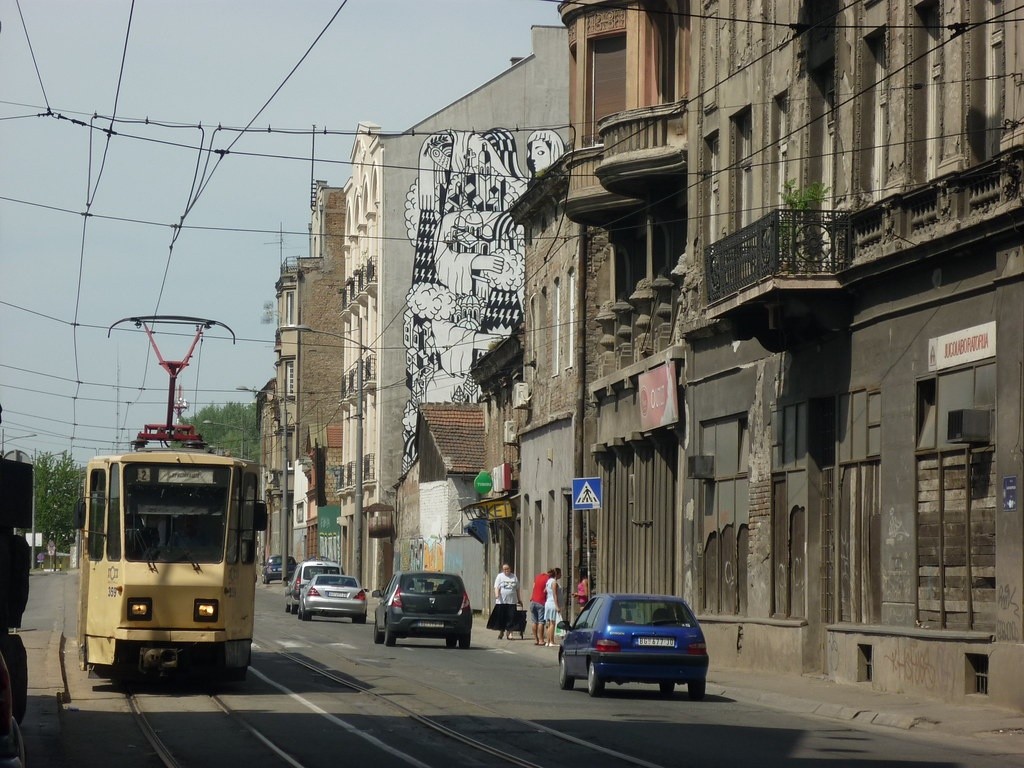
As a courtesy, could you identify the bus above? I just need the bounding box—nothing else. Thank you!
[66,313,274,699]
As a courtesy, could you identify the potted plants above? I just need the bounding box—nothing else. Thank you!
[778,178,832,272]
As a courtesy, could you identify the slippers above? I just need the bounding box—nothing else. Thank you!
[535,642,538,645]
[539,642,547,646]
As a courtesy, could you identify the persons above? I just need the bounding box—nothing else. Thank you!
[530,569,553,646]
[571,565,589,612]
[544,568,563,647]
[37,552,45,564]
[486,563,522,639]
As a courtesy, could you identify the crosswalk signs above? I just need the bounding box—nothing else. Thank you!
[572,476,604,511]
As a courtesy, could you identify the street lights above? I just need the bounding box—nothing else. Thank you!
[276,324,375,589]
[233,385,300,594]
[203,419,247,459]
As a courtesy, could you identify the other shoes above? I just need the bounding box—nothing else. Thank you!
[498,633,504,639]
[549,643,560,647]
[545,642,550,646]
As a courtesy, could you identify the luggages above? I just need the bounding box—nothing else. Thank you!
[507,602,527,639]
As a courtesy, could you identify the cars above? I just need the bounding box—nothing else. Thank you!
[555,591,710,699]
[295,574,371,623]
[371,569,474,650]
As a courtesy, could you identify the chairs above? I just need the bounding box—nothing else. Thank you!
[437,584,454,592]
[656,608,676,622]
[406,580,413,589]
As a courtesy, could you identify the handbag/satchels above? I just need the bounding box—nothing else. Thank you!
[555,611,566,637]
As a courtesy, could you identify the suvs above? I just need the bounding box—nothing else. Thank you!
[284,559,344,613]
[259,555,298,583]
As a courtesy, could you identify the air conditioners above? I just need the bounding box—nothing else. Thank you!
[504,421,518,443]
[512,383,529,409]
